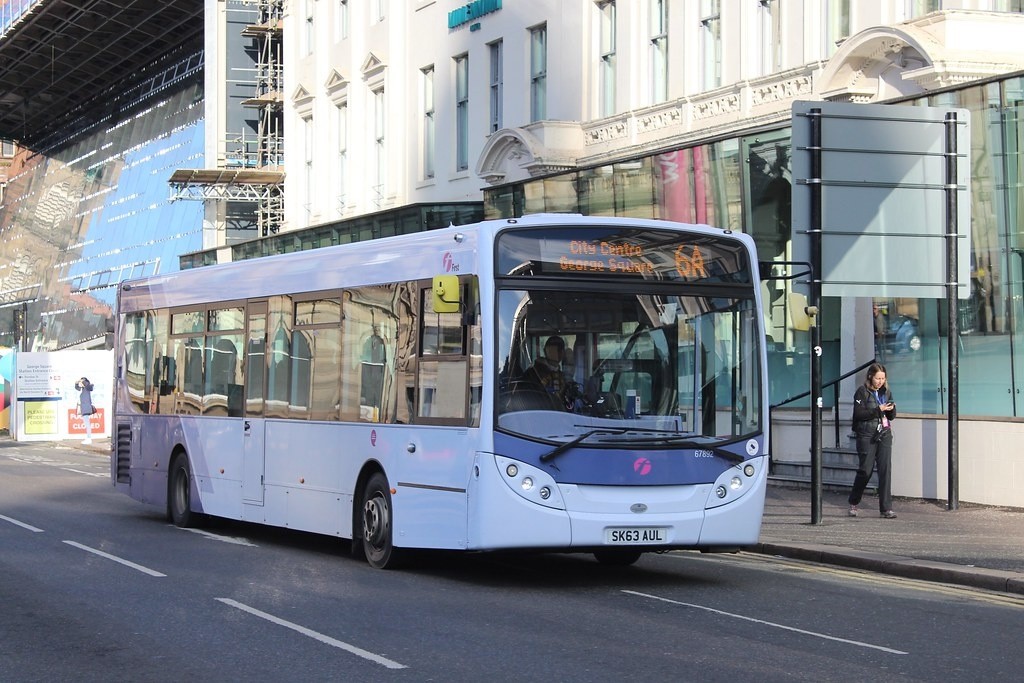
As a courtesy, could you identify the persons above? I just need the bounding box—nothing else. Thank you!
[989,269,1003,324]
[973,271,989,336]
[873,305,888,368]
[848,363,898,518]
[74,377,96,444]
[522,336,578,414]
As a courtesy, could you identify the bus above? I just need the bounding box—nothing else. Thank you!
[109,212,816,568]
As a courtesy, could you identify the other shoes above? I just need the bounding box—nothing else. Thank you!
[847,505,858,516]
[880,511,898,519]
[83,440,92,445]
